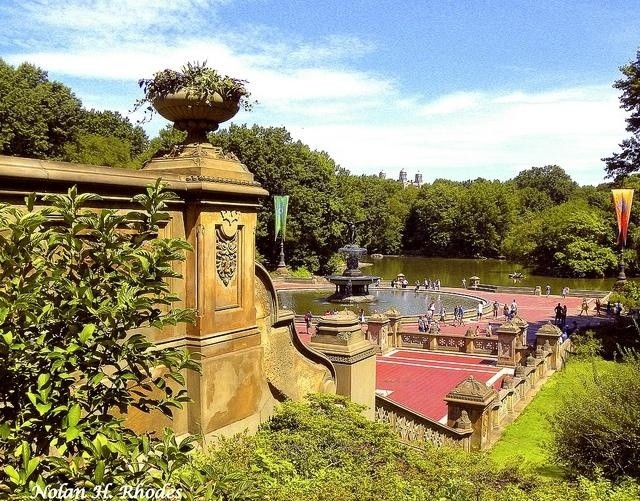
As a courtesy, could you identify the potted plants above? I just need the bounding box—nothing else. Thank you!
[127,58,262,149]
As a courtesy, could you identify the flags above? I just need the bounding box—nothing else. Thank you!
[273,195,290,243]
[611,188,635,247]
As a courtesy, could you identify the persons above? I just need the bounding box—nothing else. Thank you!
[359,309,365,322]
[562,285,573,298]
[554,302,567,326]
[391,277,408,288]
[376,279,380,287]
[544,283,551,298]
[594,298,623,318]
[305,311,313,335]
[578,298,589,317]
[461,278,467,288]
[413,278,441,291]
[417,300,500,349]
[503,299,522,321]
[324,310,339,316]
[512,271,521,278]
